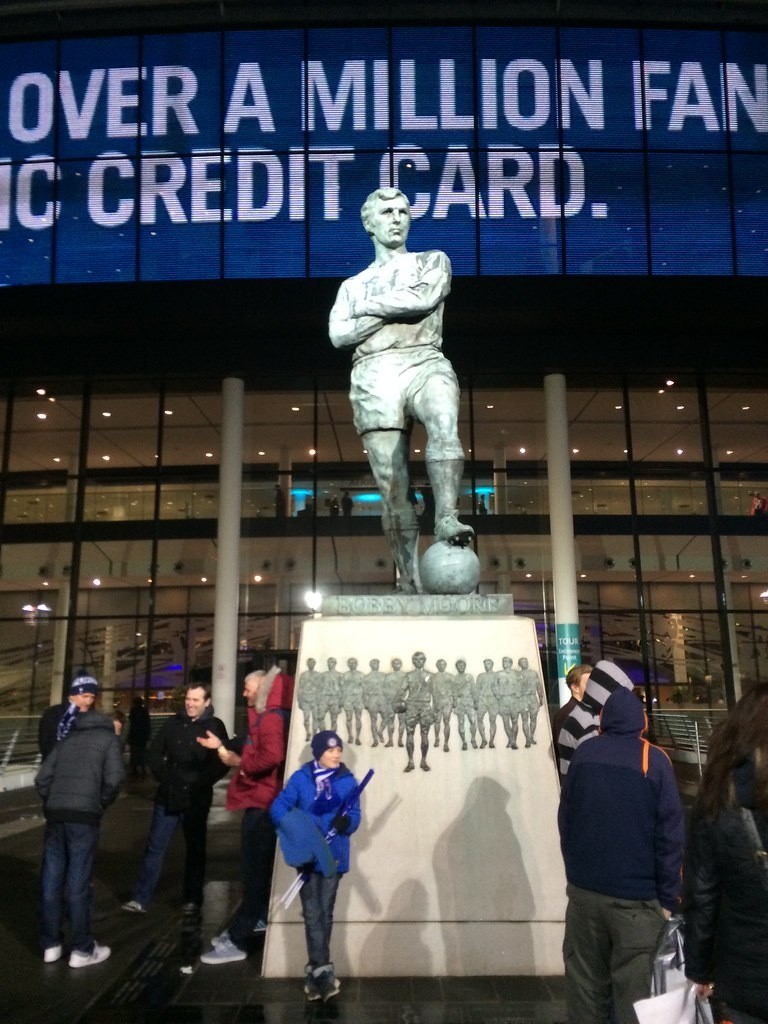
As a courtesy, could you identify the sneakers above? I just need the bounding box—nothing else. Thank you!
[305,974,321,1000]
[68,940,111,967]
[211,929,229,946]
[315,970,340,1001]
[44,945,62,962]
[201,939,247,964]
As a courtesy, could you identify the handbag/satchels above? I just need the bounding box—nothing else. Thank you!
[633,916,714,1024]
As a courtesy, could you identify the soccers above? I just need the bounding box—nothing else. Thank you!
[419,540,481,594]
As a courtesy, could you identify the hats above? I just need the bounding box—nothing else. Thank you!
[66,669,98,694]
[312,730,344,761]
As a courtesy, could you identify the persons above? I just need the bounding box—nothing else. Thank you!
[751,491,768,516]
[121,681,233,914]
[341,491,353,516]
[559,660,635,786]
[275,484,285,517]
[39,669,112,921]
[479,495,487,514]
[329,187,473,595]
[679,680,768,1024]
[270,730,361,1002]
[109,703,125,733]
[126,697,151,776]
[330,495,339,515]
[196,664,294,964]
[557,686,685,1024]
[34,710,126,968]
[552,665,592,750]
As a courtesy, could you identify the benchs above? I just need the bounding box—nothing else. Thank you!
[0,725,43,793]
[646,713,708,781]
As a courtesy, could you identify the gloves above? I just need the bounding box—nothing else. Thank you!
[332,816,351,834]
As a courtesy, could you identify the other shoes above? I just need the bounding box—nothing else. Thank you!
[181,902,200,914]
[121,900,146,912]
